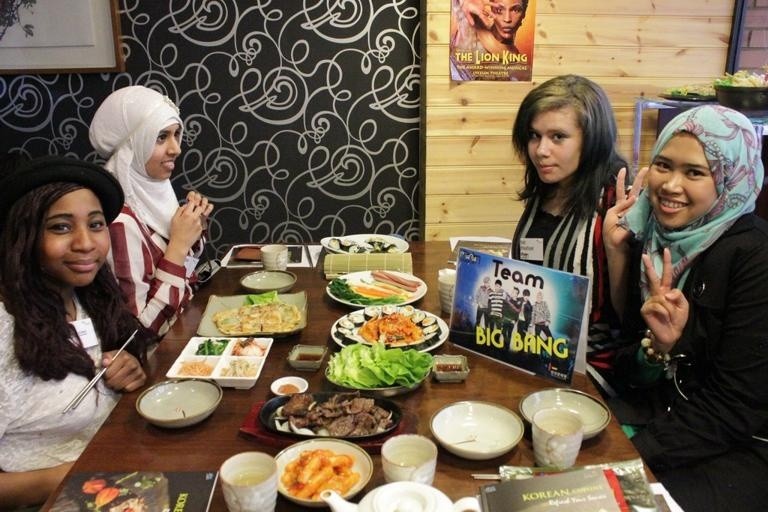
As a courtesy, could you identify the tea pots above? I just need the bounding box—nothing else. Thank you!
[321,480,481,512]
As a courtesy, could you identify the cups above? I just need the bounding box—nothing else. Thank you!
[436,277,453,314]
[530,407,584,471]
[382,434,437,486]
[260,243,287,273]
[220,449,278,512]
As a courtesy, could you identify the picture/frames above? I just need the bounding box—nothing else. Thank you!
[0,0,126,74]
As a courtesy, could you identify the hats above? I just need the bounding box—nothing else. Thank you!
[0,155,124,226]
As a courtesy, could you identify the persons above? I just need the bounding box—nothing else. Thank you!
[1,158,147,512]
[452,0,530,58]
[88,85,216,359]
[475,274,554,339]
[603,99,767,512]
[506,74,645,425]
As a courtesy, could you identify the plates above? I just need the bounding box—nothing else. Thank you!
[329,306,449,355]
[271,376,308,396]
[324,367,433,401]
[197,290,310,338]
[164,334,274,392]
[258,394,402,437]
[433,353,472,382]
[319,233,410,254]
[657,92,717,101]
[287,346,329,372]
[326,269,428,310]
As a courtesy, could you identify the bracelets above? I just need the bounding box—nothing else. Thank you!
[639,325,674,365]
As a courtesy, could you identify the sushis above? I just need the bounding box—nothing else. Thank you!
[329,237,399,253]
[334,303,442,351]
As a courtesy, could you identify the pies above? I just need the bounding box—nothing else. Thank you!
[212,302,301,334]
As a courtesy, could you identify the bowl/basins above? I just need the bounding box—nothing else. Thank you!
[715,86,768,112]
[430,399,525,462]
[273,437,374,507]
[521,387,613,441]
[133,376,223,430]
[239,270,298,295]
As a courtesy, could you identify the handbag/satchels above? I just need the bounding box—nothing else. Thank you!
[197,259,222,283]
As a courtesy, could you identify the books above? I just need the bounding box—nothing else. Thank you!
[477,456,683,512]
[46,469,222,512]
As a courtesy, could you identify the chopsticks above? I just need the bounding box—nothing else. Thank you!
[61,328,140,415]
[303,242,314,270]
